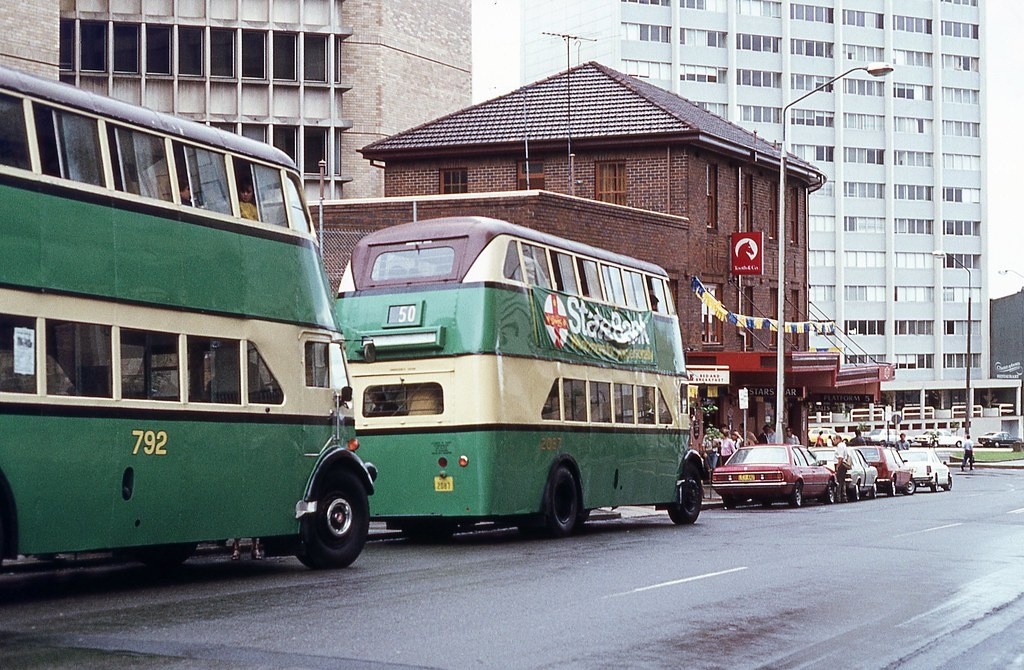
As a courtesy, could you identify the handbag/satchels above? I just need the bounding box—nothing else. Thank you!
[842,455,852,469]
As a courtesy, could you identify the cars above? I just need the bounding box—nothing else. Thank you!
[806,426,852,445]
[978,432,1022,448]
[896,449,954,493]
[863,429,915,448]
[808,447,879,502]
[914,429,966,447]
[712,445,838,509]
[851,445,917,499]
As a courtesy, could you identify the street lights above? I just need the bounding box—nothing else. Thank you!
[931,250,972,439]
[774,61,894,443]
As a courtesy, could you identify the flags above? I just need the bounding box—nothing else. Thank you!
[688,279,841,354]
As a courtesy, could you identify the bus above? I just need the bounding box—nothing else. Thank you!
[0,66,380,573]
[333,215,709,541]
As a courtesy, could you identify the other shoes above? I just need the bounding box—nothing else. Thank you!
[962,467,964,471]
[970,467,973,470]
[252,548,262,560]
[232,547,241,560]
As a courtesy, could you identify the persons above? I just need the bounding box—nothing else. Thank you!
[832,435,849,503]
[237,179,257,220]
[701,423,801,483]
[164,174,195,207]
[234,537,262,562]
[816,429,910,451]
[649,293,659,312]
[961,434,974,471]
